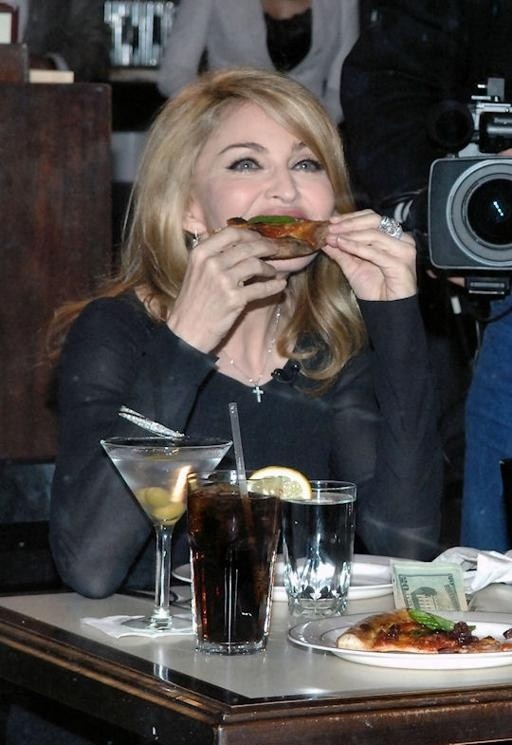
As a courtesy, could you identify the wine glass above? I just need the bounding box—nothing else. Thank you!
[98,433,233,630]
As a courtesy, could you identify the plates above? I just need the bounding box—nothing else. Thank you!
[286,611,511,674]
[169,560,399,600]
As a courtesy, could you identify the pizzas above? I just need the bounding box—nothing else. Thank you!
[335,606,509,651]
[227,214,330,259]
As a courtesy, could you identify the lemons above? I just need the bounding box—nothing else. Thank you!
[245,466,311,502]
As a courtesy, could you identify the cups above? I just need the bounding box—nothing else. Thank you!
[274,481,357,616]
[183,469,283,654]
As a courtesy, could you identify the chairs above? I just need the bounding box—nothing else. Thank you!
[1,455,57,592]
